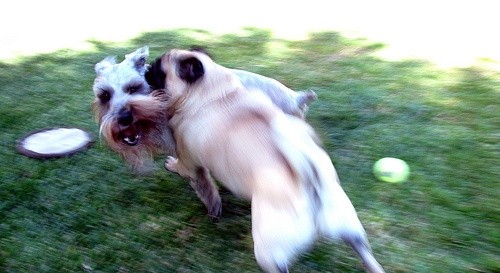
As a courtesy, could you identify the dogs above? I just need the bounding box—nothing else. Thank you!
[92,45,318,224]
[144,48,387,273]
